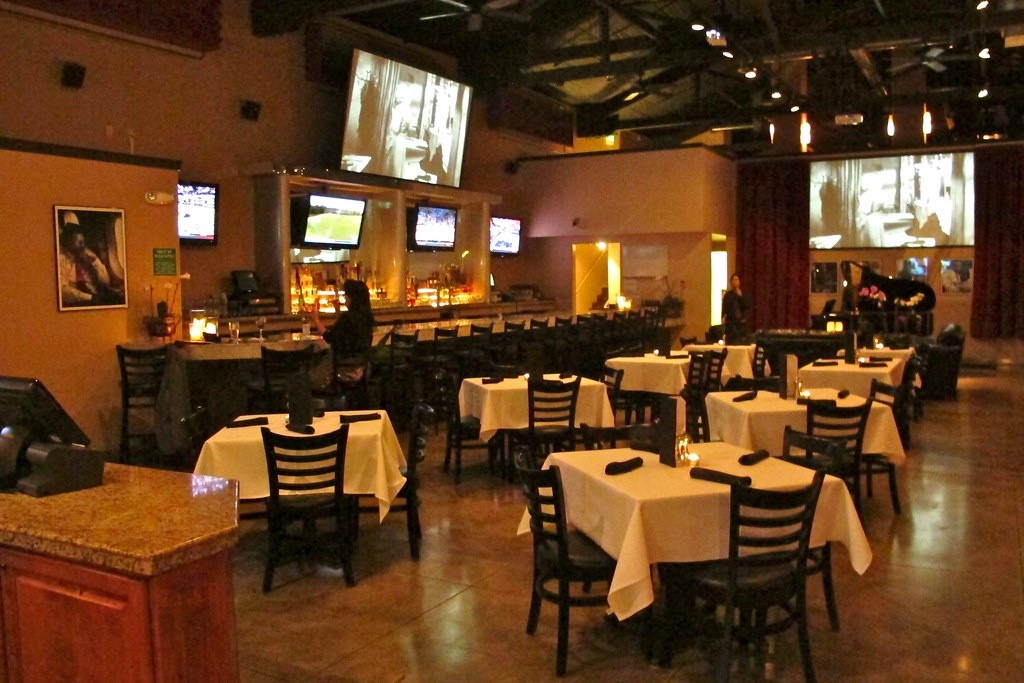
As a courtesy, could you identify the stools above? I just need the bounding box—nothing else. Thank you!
[116,308,664,462]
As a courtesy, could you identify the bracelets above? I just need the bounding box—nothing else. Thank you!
[314,320,319,325]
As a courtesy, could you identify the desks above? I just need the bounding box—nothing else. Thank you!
[797,356,906,406]
[704,387,906,466]
[605,355,731,424]
[856,345,917,361]
[681,344,771,387]
[194,411,408,557]
[516,442,873,654]
[458,373,615,482]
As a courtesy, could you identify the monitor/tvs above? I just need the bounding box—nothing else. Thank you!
[177,180,219,246]
[298,192,368,249]
[336,44,474,189]
[232,270,258,291]
[489,217,522,256]
[411,203,459,252]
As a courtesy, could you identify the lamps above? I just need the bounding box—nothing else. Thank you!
[685,16,800,113]
[972,0,991,99]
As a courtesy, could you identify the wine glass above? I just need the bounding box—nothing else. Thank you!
[256,316,267,344]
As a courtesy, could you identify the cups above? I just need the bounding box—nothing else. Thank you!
[229,321,240,345]
[302,318,311,336]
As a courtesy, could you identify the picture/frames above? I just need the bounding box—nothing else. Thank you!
[54,205,129,311]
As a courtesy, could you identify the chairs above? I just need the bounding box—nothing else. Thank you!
[258,323,930,683]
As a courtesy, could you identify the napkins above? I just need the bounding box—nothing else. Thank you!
[690,467,751,486]
[733,391,757,402]
[313,410,325,417]
[838,390,850,398]
[559,372,573,378]
[482,379,498,383]
[226,417,268,427]
[605,457,643,475]
[859,363,887,367]
[739,449,769,464]
[813,362,839,366]
[339,413,380,423]
[286,424,315,434]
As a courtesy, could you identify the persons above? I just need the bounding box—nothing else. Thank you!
[355,74,382,174]
[721,274,752,345]
[942,261,972,293]
[820,176,841,233]
[899,259,915,281]
[59,222,121,306]
[309,280,378,411]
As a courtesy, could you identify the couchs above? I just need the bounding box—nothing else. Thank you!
[915,323,966,402]
